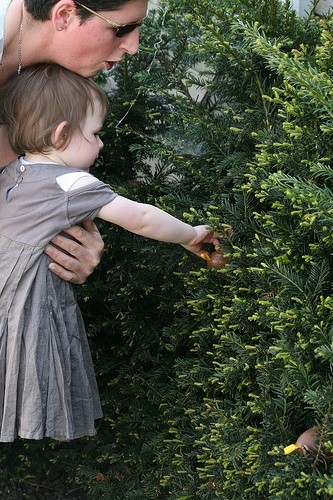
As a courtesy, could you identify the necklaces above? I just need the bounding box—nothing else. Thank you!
[17,1,26,75]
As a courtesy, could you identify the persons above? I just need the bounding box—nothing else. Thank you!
[0,64,222,442]
[0,1,150,285]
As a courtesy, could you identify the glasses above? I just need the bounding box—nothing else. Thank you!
[72,0,150,38]
